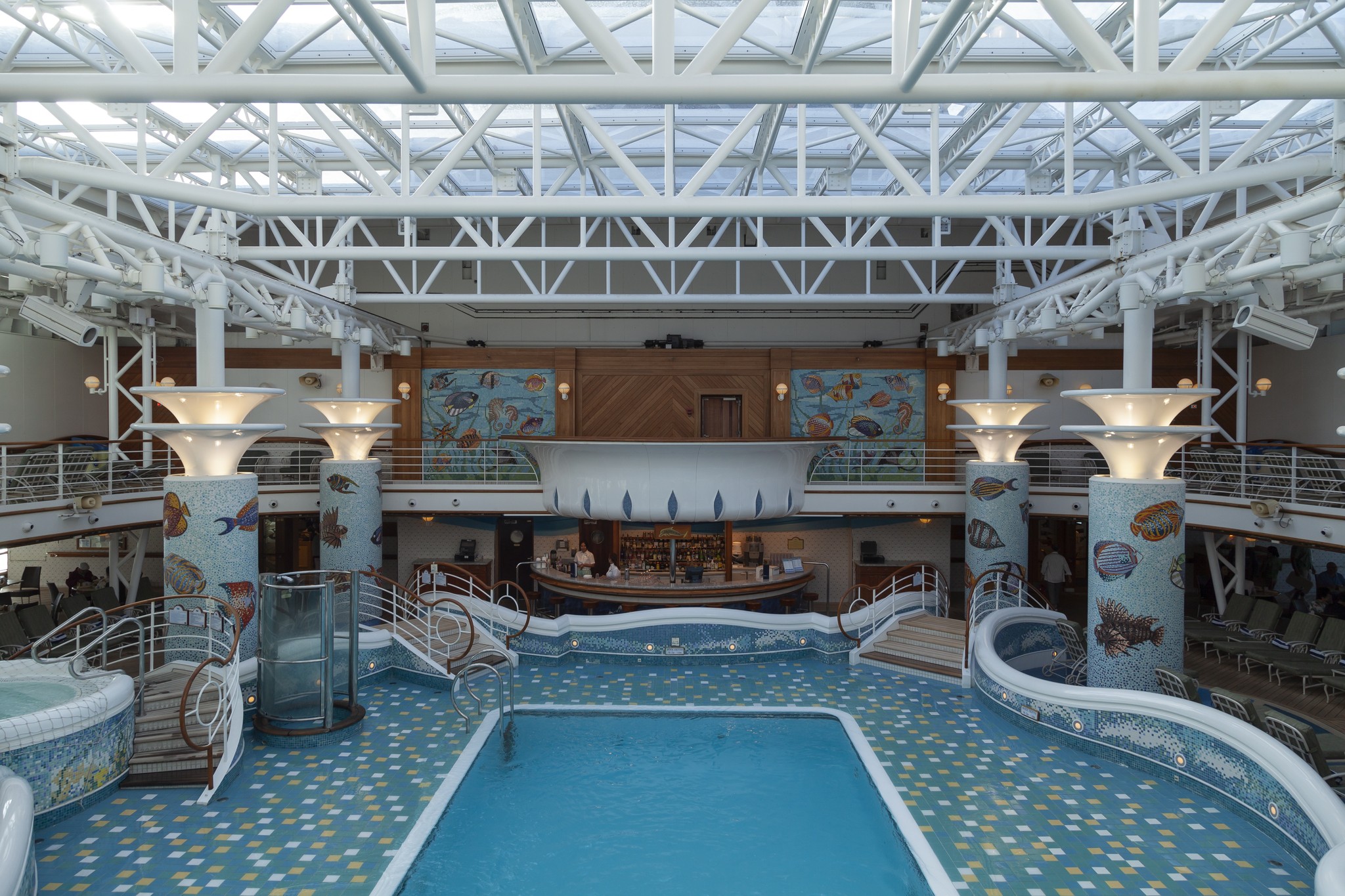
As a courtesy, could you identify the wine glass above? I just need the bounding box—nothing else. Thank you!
[640,568,658,585]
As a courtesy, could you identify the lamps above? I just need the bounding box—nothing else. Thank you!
[160,377,176,387]
[1177,378,1193,388]
[1080,384,1092,389]
[558,383,571,400]
[1007,384,1012,398]
[398,382,411,400]
[1246,538,1256,547]
[919,518,931,529]
[422,516,434,527]
[775,384,789,401]
[7,231,414,356]
[84,376,100,394]
[938,383,950,401]
[1255,378,1271,396]
[936,225,1345,357]
[336,383,342,397]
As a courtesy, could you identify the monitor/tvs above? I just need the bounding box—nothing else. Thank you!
[685,566,703,580]
[459,539,476,552]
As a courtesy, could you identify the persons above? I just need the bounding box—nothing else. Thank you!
[605,552,621,579]
[1187,529,1345,637]
[573,539,596,579]
[65,562,99,597]
[1041,543,1073,611]
[91,566,110,615]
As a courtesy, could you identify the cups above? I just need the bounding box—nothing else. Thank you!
[595,573,600,580]
[769,551,795,574]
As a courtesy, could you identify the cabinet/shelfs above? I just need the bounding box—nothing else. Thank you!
[411,557,492,605]
[854,558,936,609]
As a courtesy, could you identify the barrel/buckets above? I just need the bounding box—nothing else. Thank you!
[732,542,742,558]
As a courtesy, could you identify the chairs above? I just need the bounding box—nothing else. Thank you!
[1264,708,1345,801]
[1185,572,1345,702]
[1020,452,1062,487]
[1155,666,1204,706]
[1163,446,1345,509]
[1041,619,1088,686]
[0,567,165,667]
[1083,452,1110,487]
[1209,687,1345,760]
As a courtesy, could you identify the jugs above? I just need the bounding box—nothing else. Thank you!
[544,552,549,560]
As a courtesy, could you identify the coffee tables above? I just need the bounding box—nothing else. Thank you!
[1061,469,1086,487]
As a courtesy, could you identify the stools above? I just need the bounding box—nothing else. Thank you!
[524,591,819,620]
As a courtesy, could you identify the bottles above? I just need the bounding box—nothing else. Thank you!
[624,568,629,581]
[621,531,726,572]
